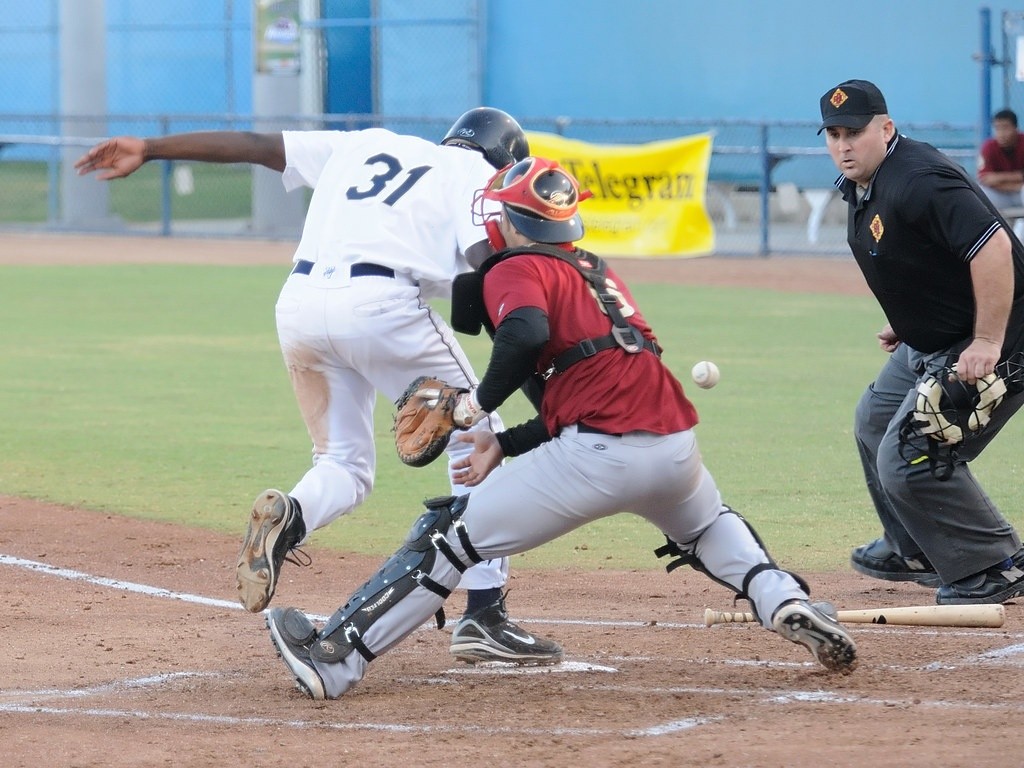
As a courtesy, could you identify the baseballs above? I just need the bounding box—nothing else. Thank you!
[691,361,720,390]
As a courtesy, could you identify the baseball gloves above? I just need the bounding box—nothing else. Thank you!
[393,375,470,468]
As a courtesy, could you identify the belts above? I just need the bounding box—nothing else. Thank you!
[292,260,396,280]
[577,422,622,437]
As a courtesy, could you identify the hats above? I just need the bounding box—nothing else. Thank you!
[817,79,888,136]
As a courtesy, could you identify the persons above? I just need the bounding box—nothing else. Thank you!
[75,106,563,667]
[975,109,1024,245]
[817,80,1024,605]
[265,157,859,700]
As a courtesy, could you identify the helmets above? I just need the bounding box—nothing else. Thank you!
[482,155,585,244]
[440,107,530,171]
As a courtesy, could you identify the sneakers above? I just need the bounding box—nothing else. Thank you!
[851,538,943,588]
[450,588,563,665]
[935,548,1024,605]
[771,599,858,672]
[264,607,327,701]
[235,489,312,614]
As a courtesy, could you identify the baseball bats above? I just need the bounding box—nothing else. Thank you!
[704,603,1006,628]
